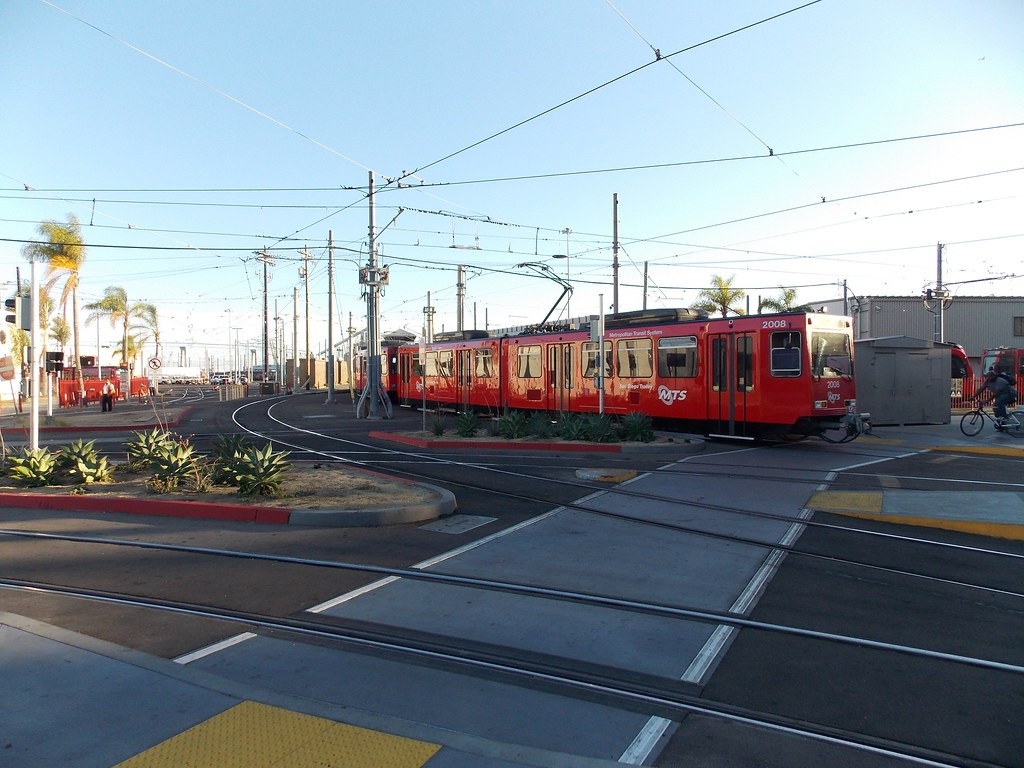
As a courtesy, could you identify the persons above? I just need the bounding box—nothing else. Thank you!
[969,369,1018,428]
[101,378,115,412]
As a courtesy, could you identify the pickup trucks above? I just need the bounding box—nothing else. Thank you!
[157,375,199,385]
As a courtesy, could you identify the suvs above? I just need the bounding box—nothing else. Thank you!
[210,375,247,385]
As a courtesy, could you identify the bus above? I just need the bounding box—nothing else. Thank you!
[354,261,882,446]
[980,345,1024,406]
[946,340,976,403]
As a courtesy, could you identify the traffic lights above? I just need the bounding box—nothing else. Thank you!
[6,297,30,331]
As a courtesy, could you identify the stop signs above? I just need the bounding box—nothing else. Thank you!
[0,355,16,382]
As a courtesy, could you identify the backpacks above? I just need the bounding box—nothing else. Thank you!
[995,372,1017,386]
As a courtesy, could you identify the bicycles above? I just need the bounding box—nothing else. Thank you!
[959,397,1024,438]
